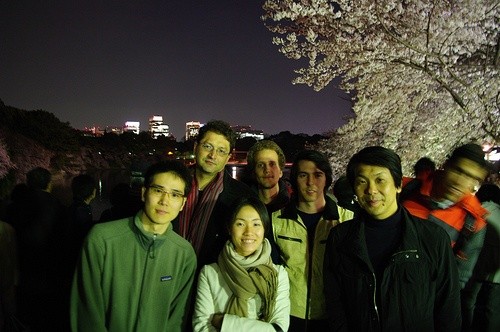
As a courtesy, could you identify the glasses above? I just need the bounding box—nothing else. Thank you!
[199,143,229,156]
[146,185,186,201]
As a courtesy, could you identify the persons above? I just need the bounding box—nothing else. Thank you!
[158,120,252,266]
[322,143,499,332]
[0,164,147,332]
[225,140,299,223]
[70,164,199,332]
[192,197,292,332]
[323,146,462,332]
[272,151,359,332]
